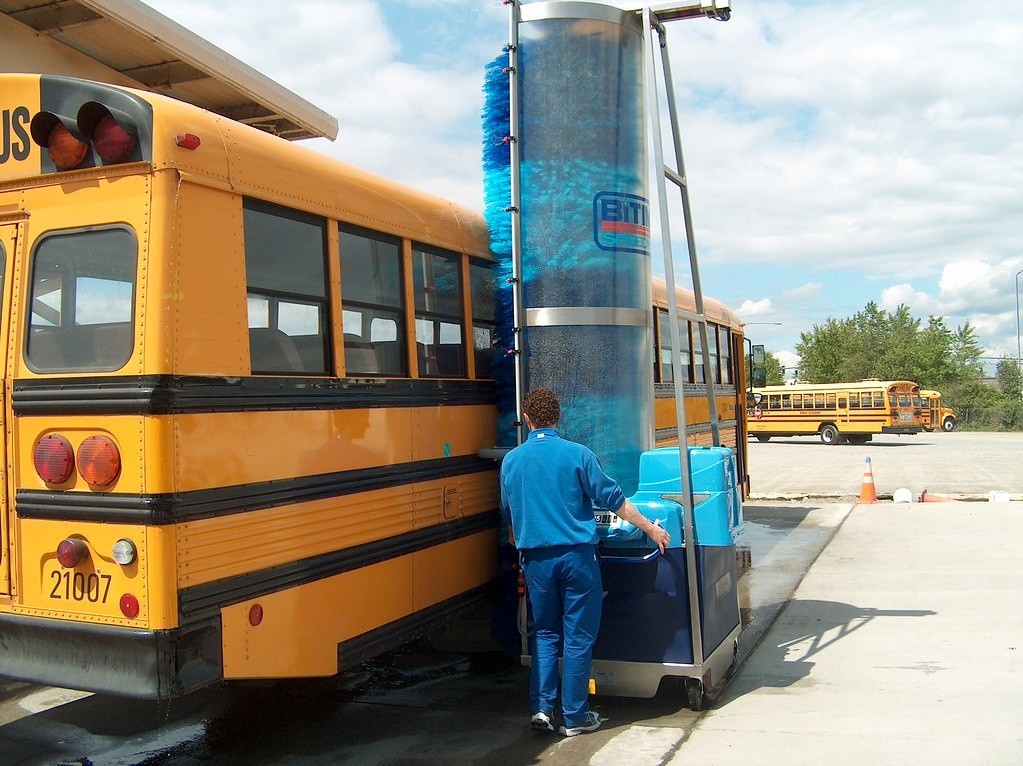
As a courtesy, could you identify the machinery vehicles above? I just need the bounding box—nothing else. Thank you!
[918,389,956,434]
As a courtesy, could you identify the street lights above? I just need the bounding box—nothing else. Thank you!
[1016,270,1023,375]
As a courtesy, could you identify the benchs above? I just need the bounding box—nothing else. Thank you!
[425,343,465,376]
[247,326,306,374]
[475,348,493,378]
[371,340,439,378]
[289,333,382,375]
[32,321,133,373]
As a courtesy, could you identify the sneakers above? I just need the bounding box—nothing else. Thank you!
[529,712,555,734]
[558,710,600,736]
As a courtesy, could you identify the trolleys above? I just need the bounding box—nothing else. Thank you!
[511,444,747,711]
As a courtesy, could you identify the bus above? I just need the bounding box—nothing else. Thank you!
[0,71,766,707]
[745,378,923,445]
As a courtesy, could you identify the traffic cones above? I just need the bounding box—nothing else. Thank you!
[916,490,961,502]
[854,456,879,504]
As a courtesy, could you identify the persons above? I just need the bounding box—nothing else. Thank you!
[497,388,671,737]
